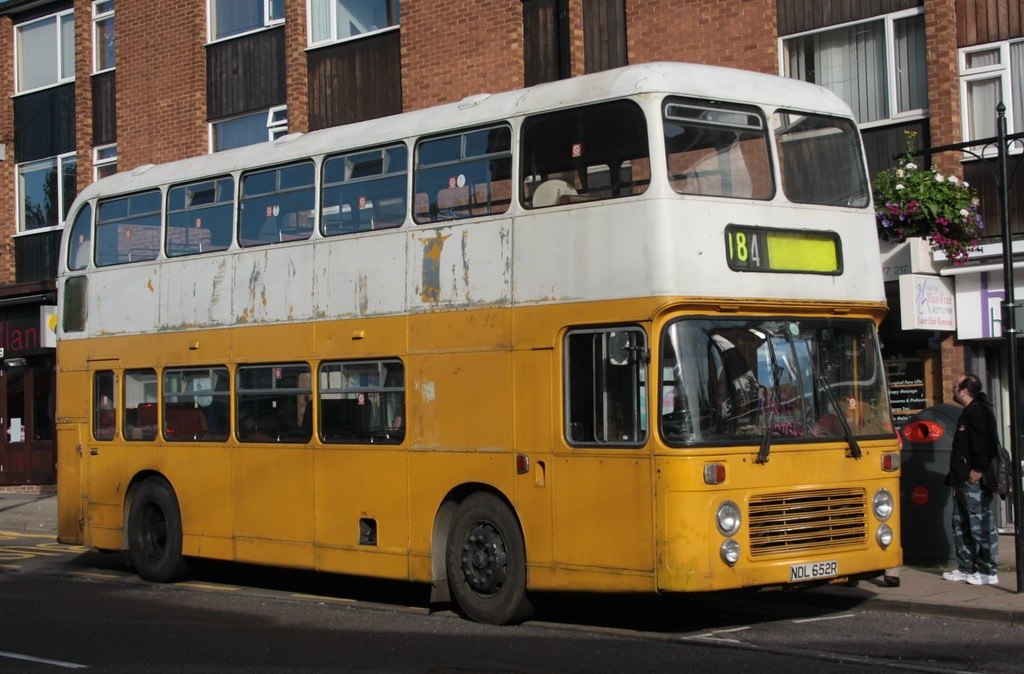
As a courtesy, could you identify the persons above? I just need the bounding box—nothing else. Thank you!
[943,373,999,584]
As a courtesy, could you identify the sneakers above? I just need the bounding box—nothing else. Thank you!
[965,571,998,585]
[943,569,972,581]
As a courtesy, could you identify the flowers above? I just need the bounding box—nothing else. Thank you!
[870,157,986,268]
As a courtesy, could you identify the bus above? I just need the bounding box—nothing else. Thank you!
[52,60,907,628]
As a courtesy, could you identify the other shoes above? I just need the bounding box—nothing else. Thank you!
[884,576,900,586]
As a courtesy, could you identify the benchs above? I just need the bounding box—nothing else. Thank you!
[95,397,406,447]
[124,217,344,264]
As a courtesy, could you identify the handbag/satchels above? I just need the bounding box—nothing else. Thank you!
[992,443,1013,500]
